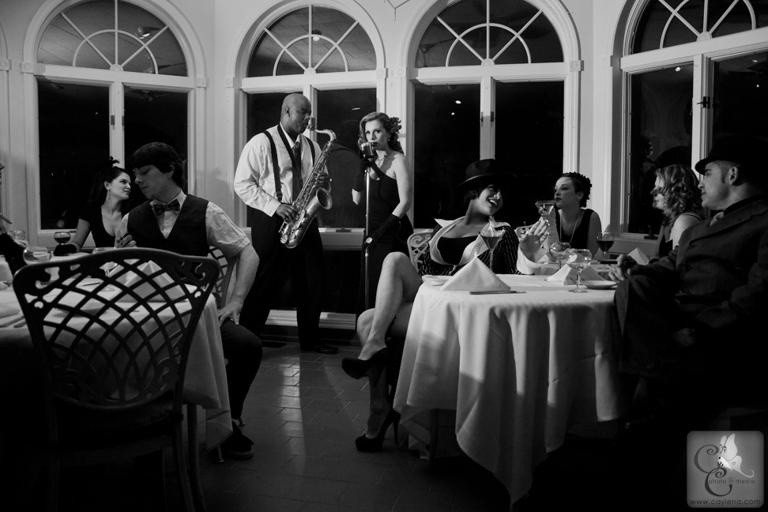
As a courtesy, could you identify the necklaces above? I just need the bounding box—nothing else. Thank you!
[555,211,581,244]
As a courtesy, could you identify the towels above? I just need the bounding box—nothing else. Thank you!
[441,257,511,292]
[627,247,649,265]
[547,256,606,285]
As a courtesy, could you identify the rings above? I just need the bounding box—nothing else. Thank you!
[535,234,541,240]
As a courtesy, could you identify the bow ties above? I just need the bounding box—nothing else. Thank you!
[150,198,183,217]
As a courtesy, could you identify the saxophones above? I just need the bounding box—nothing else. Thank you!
[278,125,336,248]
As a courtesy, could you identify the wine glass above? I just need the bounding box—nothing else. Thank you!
[91,246,118,292]
[7,227,73,286]
[478,231,504,271]
[515,200,616,294]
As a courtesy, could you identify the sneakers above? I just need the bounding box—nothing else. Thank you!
[224,422,259,461]
[340,347,403,380]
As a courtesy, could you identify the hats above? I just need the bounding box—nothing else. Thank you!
[692,135,768,175]
[454,157,520,190]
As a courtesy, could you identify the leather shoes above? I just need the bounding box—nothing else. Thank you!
[316,343,339,354]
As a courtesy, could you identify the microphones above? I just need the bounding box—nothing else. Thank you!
[360,141,379,154]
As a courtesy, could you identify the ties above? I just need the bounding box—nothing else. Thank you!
[291,148,301,204]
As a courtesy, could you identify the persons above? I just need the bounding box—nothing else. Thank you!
[233,92,345,356]
[535,172,603,260]
[646,159,705,259]
[51,166,140,254]
[341,157,549,454]
[604,132,768,435]
[346,110,416,337]
[0,158,51,288]
[113,142,266,460]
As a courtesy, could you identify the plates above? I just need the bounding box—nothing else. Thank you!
[59,302,108,312]
[419,274,453,284]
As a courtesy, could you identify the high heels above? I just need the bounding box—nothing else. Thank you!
[355,408,401,452]
